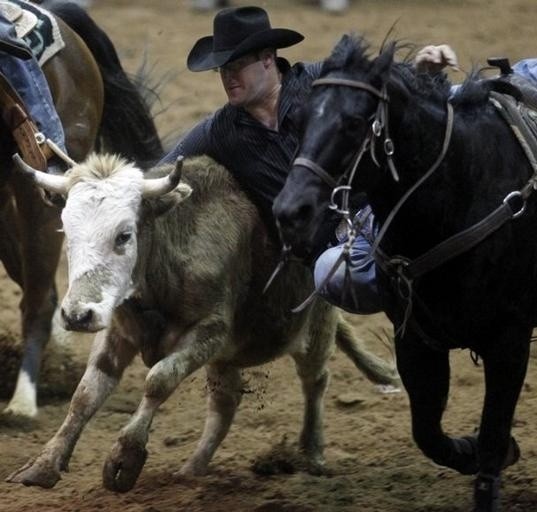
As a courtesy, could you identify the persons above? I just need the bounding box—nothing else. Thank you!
[0,10,66,200]
[139,7,459,313]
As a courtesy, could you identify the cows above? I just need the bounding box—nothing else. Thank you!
[3,150,404,494]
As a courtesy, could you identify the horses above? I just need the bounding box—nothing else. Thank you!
[0,0,165,425]
[272,19,537,512]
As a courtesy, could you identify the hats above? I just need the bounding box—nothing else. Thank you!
[186,5,305,72]
